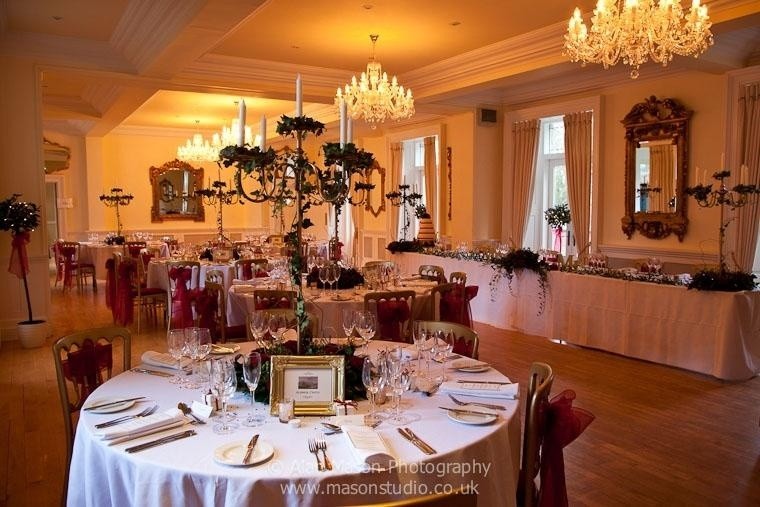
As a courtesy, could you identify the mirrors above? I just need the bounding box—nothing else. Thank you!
[618,95,696,239]
[148,156,207,222]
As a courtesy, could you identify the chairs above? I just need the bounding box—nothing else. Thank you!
[47,227,594,507]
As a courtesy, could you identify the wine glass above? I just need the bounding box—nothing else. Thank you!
[526,246,563,271]
[165,309,455,431]
[252,257,434,301]
[640,254,665,278]
[582,254,608,274]
[424,233,512,261]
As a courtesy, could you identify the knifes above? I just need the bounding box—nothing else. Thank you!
[82,396,146,410]
[457,379,512,386]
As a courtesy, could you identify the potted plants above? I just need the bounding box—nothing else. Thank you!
[1,192,47,348]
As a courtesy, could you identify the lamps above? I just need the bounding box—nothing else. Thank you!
[561,0,716,79]
[211,101,263,158]
[335,32,415,130]
[174,119,220,163]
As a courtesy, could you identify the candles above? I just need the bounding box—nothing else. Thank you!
[337,98,347,149]
[347,117,353,144]
[702,168,708,186]
[695,166,701,185]
[740,164,745,184]
[721,152,725,170]
[237,98,247,146]
[260,114,267,151]
[228,177,232,190]
[217,167,221,181]
[208,175,210,189]
[295,70,304,116]
[743,166,750,185]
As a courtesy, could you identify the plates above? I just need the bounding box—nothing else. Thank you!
[215,355,496,474]
